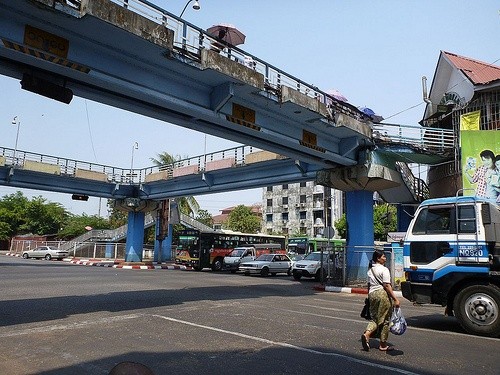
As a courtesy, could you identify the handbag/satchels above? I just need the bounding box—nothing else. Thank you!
[389,306,407,336]
[360,297,371,321]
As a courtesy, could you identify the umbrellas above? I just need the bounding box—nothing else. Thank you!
[206,24,246,48]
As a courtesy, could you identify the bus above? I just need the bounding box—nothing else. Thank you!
[174,227,286,271]
[285,237,346,259]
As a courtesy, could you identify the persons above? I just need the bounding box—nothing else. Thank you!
[210,30,225,54]
[361,251,400,352]
[465,150,500,207]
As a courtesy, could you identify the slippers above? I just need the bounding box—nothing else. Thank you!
[379,346,394,350]
[361,334,369,351]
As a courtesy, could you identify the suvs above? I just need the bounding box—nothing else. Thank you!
[292,251,340,281]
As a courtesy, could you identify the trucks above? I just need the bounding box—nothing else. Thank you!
[381,188,500,336]
[222,247,257,273]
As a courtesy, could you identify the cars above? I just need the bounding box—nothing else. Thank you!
[22,246,69,261]
[237,254,296,278]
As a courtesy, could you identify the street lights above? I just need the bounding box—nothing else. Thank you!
[131,142,139,182]
[11,115,20,165]
[175,0,200,42]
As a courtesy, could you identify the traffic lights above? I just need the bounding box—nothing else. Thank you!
[71,193,89,202]
